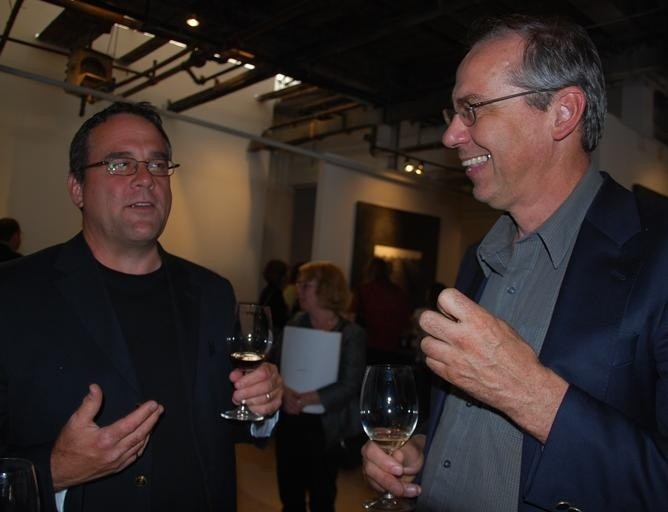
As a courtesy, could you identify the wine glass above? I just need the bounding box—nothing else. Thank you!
[358,362,420,511]
[222,302,273,424]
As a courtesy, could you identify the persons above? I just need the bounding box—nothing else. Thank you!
[0,217,23,262]
[361,13,668,512]
[250,253,445,511]
[0,99,285,512]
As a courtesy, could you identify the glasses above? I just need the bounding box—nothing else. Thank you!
[443,89,554,126]
[82,158,180,176]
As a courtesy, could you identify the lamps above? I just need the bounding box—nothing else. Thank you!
[401,156,424,176]
